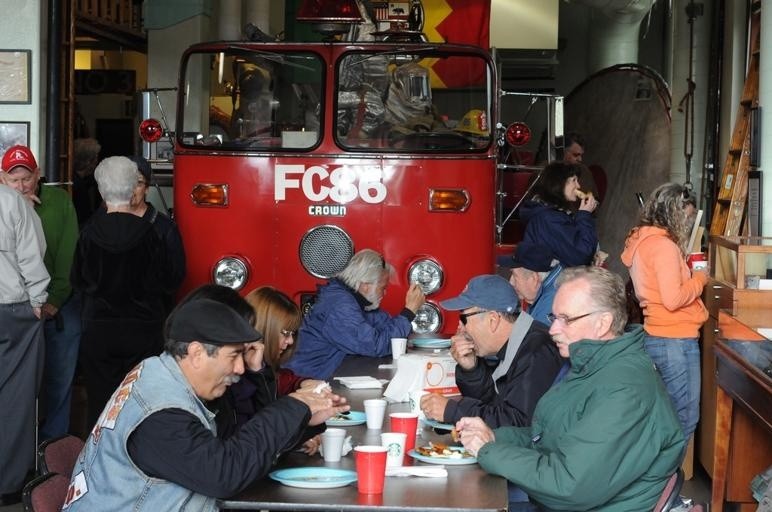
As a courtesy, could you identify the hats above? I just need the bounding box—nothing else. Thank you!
[438,274,521,315]
[164,298,263,344]
[128,155,151,180]
[497,242,560,272]
[2,144,37,173]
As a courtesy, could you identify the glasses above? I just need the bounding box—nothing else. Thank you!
[459,310,486,325]
[280,328,297,337]
[681,186,690,201]
[547,311,596,326]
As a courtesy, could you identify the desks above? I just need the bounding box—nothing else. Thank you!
[216,335,512,512]
[710,336,771,512]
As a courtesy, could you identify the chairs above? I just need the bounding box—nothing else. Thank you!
[654,465,689,512]
[23,472,71,511]
[37,434,86,481]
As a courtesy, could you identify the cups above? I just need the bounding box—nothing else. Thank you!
[591,250,608,268]
[353,444,388,494]
[357,492,383,506]
[382,431,407,466]
[686,252,708,283]
[391,337,408,359]
[390,412,418,451]
[362,399,388,429]
[321,428,347,462]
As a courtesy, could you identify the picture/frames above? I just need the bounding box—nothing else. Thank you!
[138,129,201,175]
[0,47,31,105]
[1,120,31,166]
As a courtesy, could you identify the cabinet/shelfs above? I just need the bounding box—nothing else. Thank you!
[698,236,772,504]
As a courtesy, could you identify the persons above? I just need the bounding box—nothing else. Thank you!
[514,136,716,510]
[62,248,687,510]
[0,145,188,507]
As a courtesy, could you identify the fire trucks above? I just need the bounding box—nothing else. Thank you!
[139,0,567,339]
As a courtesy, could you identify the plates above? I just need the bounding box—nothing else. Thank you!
[267,466,358,488]
[410,336,451,348]
[424,417,457,431]
[324,410,366,425]
[410,444,481,465]
